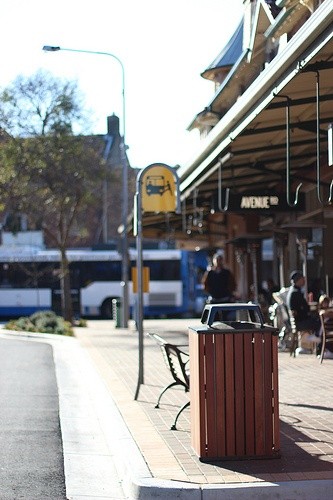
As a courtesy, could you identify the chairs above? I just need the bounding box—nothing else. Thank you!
[320,313,333,364]
[288,310,317,358]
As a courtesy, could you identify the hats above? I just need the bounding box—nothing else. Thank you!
[291,271,303,283]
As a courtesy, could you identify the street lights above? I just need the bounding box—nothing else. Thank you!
[42,45,129,328]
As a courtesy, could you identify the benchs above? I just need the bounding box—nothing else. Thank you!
[148,334,190,430]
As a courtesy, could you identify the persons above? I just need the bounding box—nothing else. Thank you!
[272,271,333,354]
[202,254,237,303]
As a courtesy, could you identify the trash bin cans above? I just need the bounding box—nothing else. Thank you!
[188,303,281,464]
[112,299,121,327]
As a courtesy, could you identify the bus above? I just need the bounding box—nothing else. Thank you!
[0,249,208,319]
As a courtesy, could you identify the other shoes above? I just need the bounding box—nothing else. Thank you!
[324,351,333,359]
